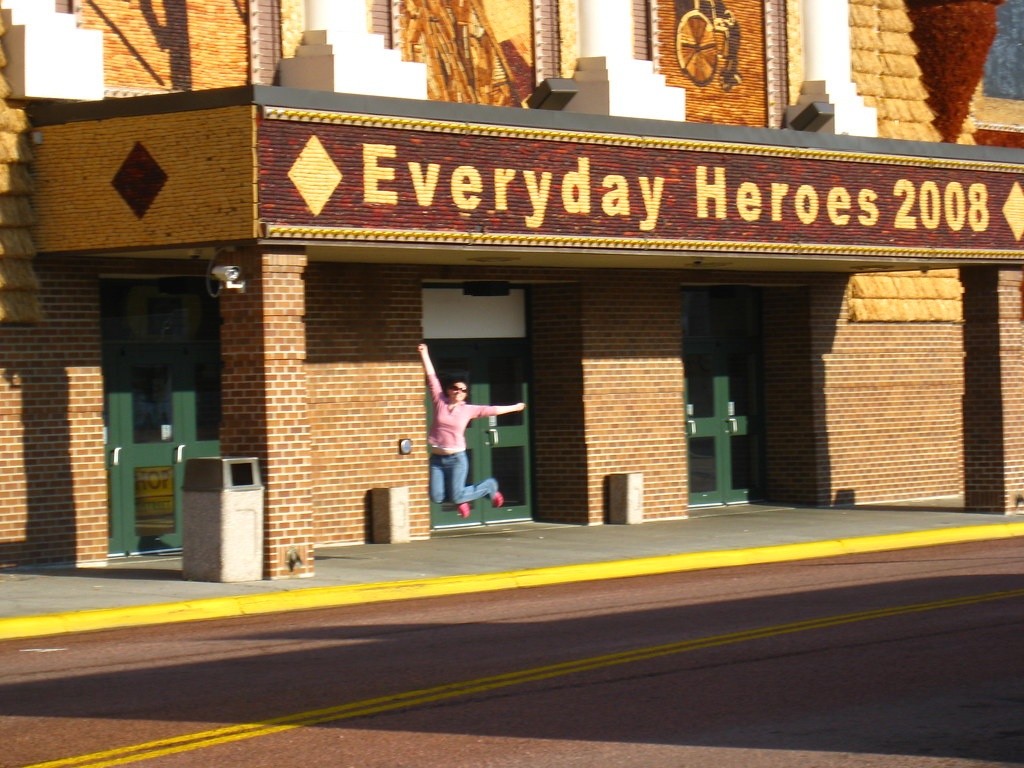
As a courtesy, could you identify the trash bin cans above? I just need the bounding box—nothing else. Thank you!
[373,487,411,544]
[608,471,644,524]
[180,457,265,584]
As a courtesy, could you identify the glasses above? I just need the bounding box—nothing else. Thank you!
[450,386,467,393]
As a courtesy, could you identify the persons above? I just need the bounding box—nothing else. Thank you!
[416,344,526,518]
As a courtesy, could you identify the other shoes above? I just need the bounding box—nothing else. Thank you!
[456,503,470,518]
[492,491,504,508]
